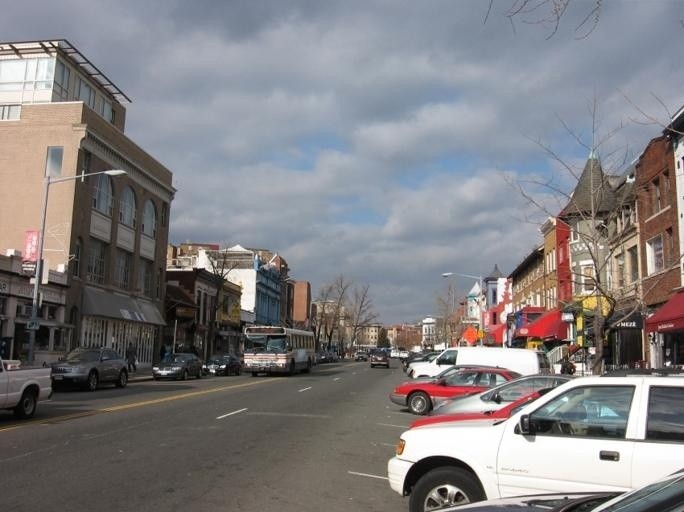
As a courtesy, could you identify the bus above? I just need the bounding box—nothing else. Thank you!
[242,324,315,378]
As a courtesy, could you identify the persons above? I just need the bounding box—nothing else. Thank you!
[125,343,137,373]
[561,357,576,374]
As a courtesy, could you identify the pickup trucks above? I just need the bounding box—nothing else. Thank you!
[0,356,55,418]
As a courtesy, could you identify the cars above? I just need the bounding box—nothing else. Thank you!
[429,375,618,418]
[409,388,630,431]
[424,469,684,511]
[389,348,441,376]
[151,352,203,381]
[355,352,368,361]
[202,354,242,377]
[413,365,504,381]
[316,350,339,363]
[389,368,523,416]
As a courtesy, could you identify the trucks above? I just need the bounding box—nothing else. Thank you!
[412,346,550,382]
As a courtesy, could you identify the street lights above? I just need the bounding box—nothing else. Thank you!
[308,300,335,332]
[27,169,127,368]
[441,272,484,347]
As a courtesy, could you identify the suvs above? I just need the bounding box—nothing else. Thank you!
[370,350,389,369]
[47,346,129,392]
[387,368,684,512]
